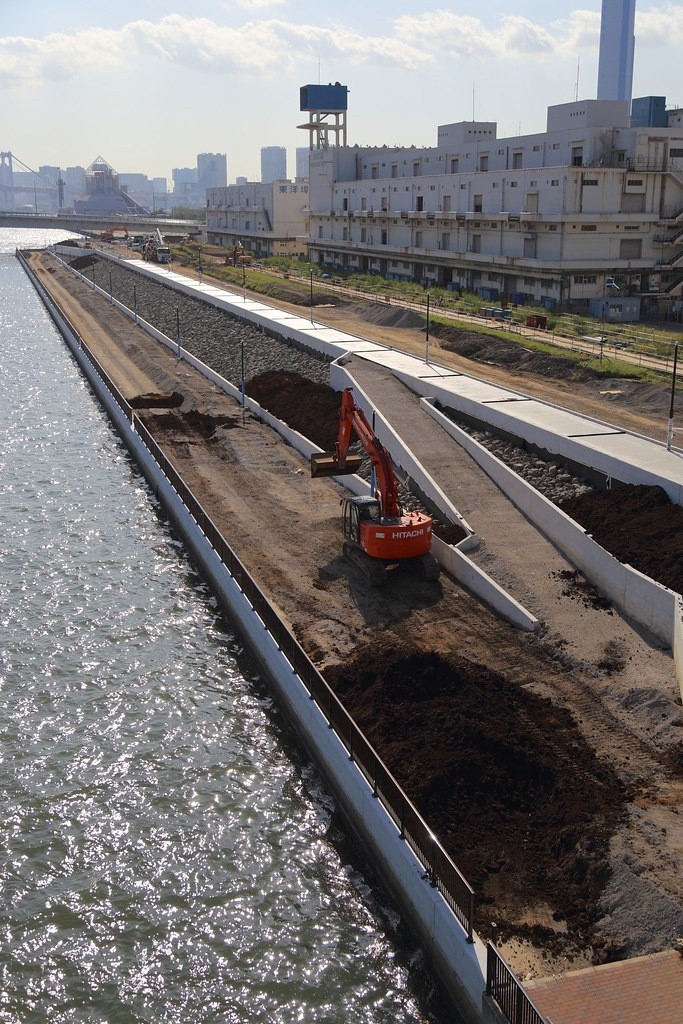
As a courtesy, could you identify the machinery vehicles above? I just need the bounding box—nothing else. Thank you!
[225,241,253,269]
[307,384,441,584]
